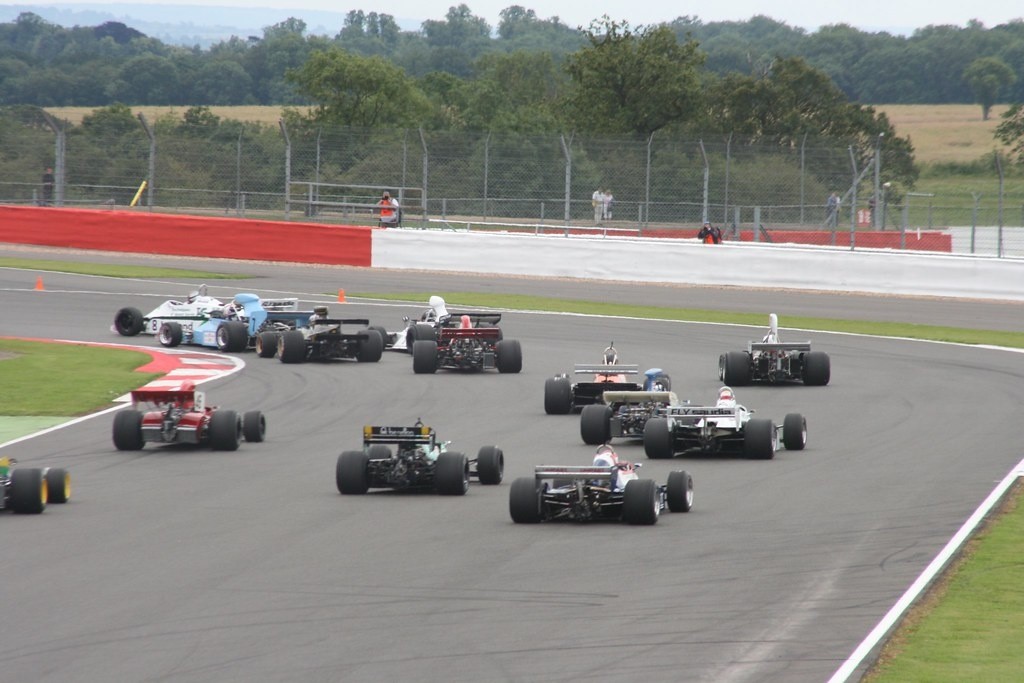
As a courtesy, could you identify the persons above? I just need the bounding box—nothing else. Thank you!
[834,190,841,228]
[602,190,615,221]
[752,333,780,360]
[42,165,55,208]
[182,291,202,305]
[378,191,400,228]
[421,308,437,323]
[586,449,638,491]
[593,353,628,384]
[592,187,604,223]
[696,389,751,432]
[697,220,719,245]
[172,379,196,411]
[868,190,882,229]
[306,305,328,330]
[825,192,837,228]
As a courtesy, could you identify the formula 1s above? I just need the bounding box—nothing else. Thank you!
[109,283,391,362]
[405,294,523,375]
[546,342,810,461]
[508,443,696,526]
[335,415,504,492]
[719,313,834,388]
[108,379,268,451]
[0,455,75,512]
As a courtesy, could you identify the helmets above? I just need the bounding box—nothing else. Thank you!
[421,311,435,322]
[309,312,330,321]
[602,353,618,364]
[592,453,615,467]
[719,391,733,401]
[460,315,470,327]
[187,292,199,303]
[762,335,779,344]
